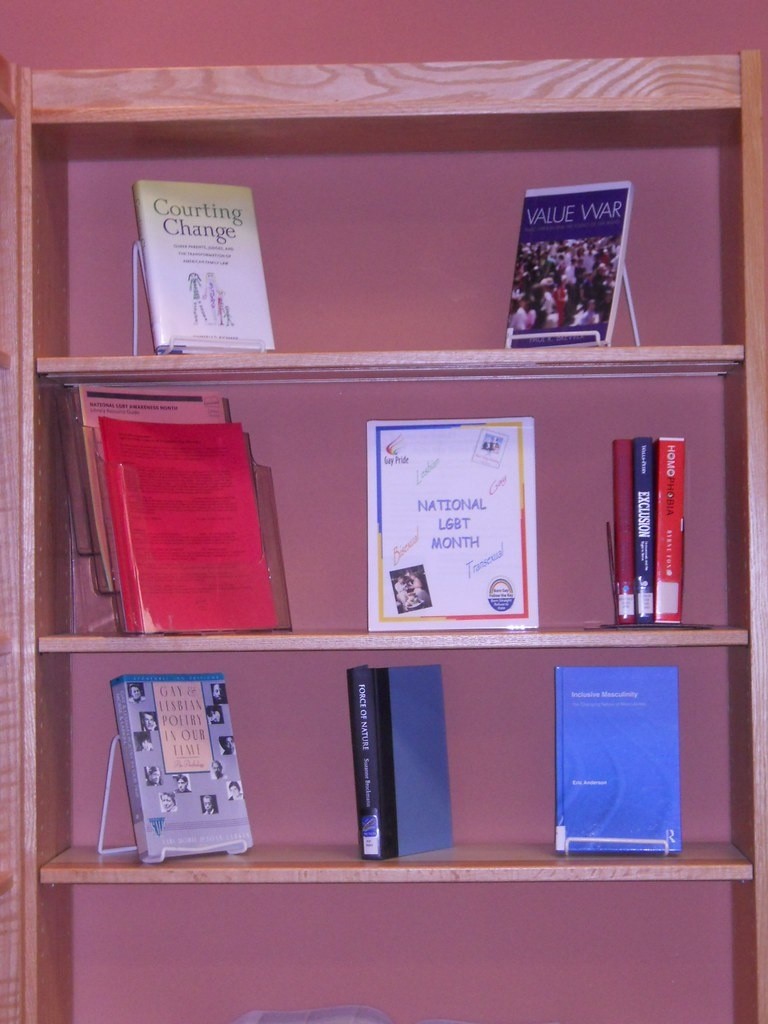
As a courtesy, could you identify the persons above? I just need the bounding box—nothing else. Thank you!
[128,683,244,815]
[507,235,622,331]
[394,574,429,611]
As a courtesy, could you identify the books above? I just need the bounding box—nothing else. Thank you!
[73,383,280,634]
[613,437,684,625]
[132,181,274,354]
[551,664,682,859]
[507,182,632,344]
[346,661,457,860]
[110,670,254,858]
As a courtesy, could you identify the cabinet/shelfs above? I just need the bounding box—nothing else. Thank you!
[0,49,768,1024]
